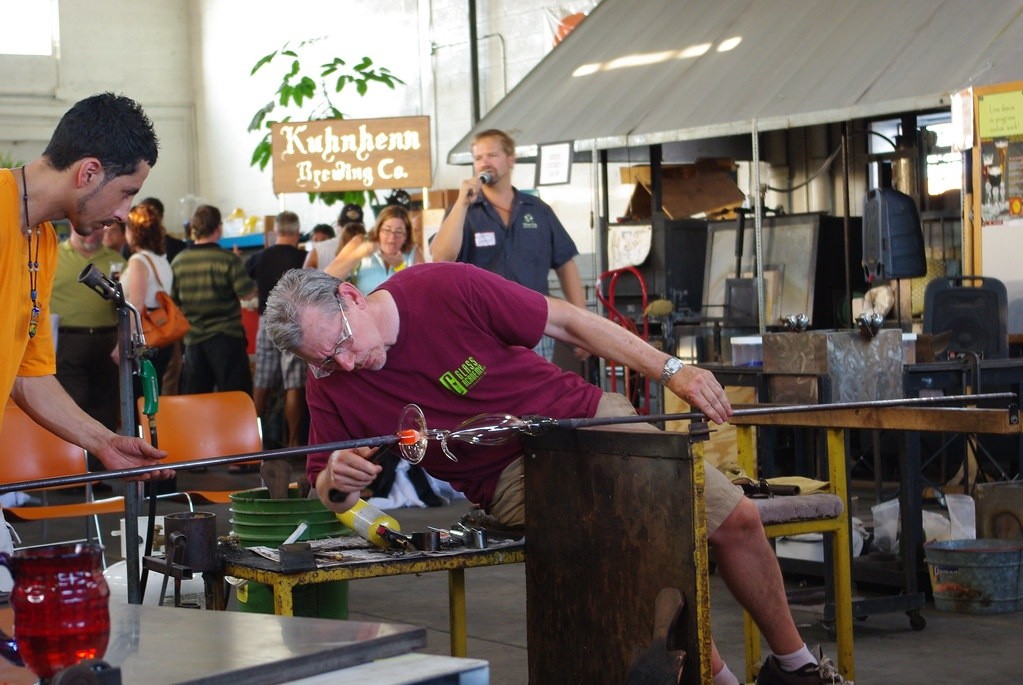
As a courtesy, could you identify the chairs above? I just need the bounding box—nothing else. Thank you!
[0,395,106,572]
[138,391,271,513]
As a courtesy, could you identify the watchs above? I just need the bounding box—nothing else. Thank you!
[657,358,683,385]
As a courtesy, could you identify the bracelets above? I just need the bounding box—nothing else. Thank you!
[393,261,407,272]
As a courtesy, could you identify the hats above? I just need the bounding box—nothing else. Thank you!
[339,203,363,227]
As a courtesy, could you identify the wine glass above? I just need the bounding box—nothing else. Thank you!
[982,140,1010,214]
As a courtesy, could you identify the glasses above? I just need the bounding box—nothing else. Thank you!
[308,292,354,379]
[381,229,407,237]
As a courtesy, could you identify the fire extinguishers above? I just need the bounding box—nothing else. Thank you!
[335,498,411,551]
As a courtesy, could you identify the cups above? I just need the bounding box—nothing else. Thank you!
[8,542,111,685]
[109,262,123,286]
[164,512,216,572]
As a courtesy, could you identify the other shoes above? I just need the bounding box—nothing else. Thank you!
[755,643,855,685]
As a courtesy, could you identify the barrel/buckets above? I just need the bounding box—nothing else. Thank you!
[228,488,350,619]
[922,539,1023,614]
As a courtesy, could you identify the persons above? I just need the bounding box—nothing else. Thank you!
[0,93,176,481]
[47,197,424,493]
[427,129,592,361]
[264,262,857,685]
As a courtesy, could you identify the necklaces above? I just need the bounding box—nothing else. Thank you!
[22,165,41,339]
[488,203,512,214]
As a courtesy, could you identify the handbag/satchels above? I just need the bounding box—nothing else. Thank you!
[141,291,192,351]
